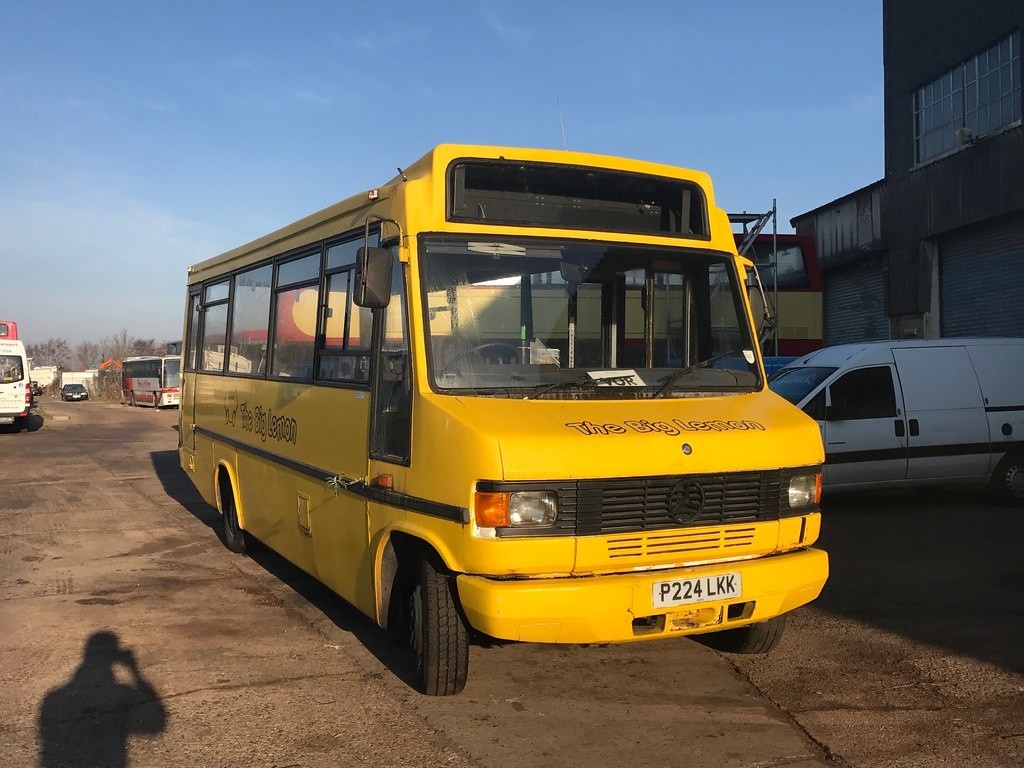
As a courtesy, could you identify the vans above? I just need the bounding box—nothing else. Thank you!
[766,335,1024,510]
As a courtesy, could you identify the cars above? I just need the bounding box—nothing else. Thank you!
[30,380,43,396]
[59,384,88,401]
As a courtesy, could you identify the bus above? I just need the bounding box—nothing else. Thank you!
[122,354,181,409]
[0,321,33,424]
[175,141,831,696]
